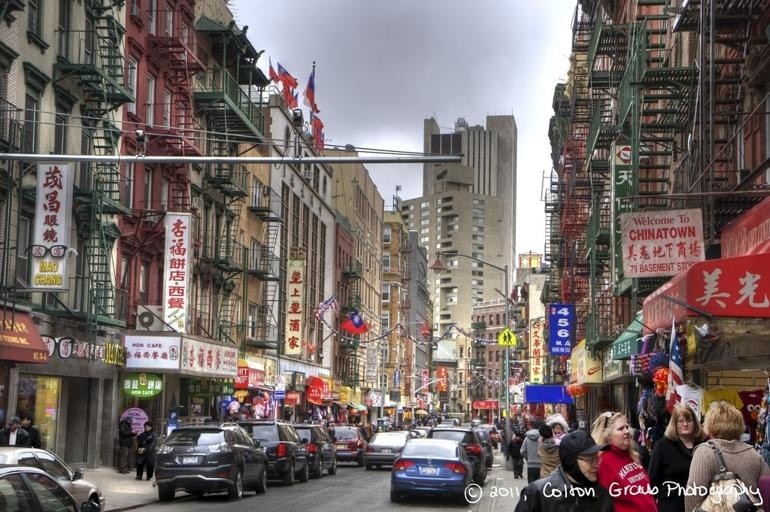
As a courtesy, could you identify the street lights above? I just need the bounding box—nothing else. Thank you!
[429,252,512,445]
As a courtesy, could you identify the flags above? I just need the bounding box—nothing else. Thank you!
[665,328,683,414]
[269,63,325,151]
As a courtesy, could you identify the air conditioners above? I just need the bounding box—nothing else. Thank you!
[136,305,163,332]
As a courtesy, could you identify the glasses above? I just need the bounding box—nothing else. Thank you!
[603,412,618,428]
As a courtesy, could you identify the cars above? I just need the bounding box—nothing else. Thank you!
[1,447,107,511]
[470,419,484,428]
[0,465,101,512]
[365,427,432,473]
[436,417,461,428]
[473,429,494,468]
[391,438,477,507]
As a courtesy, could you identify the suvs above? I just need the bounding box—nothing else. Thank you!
[289,424,339,479]
[236,419,309,487]
[477,424,499,449]
[330,425,368,466]
[156,420,271,501]
[428,428,488,487]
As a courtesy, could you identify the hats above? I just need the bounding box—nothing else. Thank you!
[558,430,610,464]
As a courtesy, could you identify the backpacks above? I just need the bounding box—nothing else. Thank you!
[696,440,763,512]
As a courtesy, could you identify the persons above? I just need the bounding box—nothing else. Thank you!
[135,421,157,480]
[118,415,136,474]
[20,415,42,448]
[489,401,770,512]
[361,413,445,439]
[0,415,31,448]
